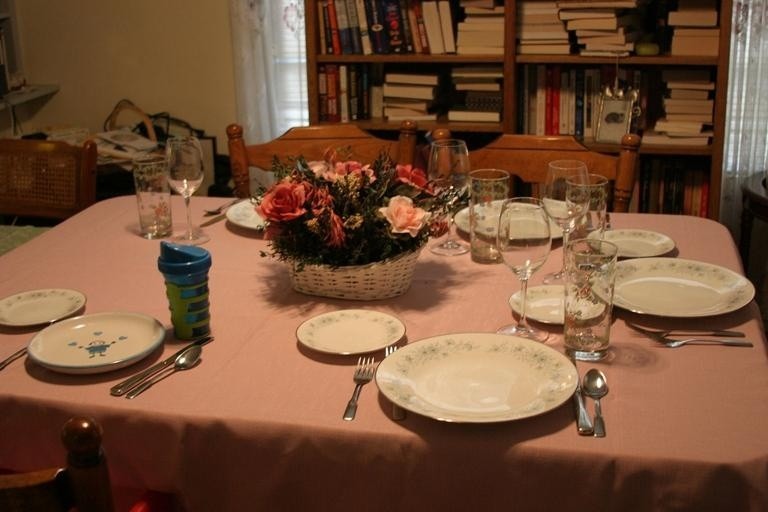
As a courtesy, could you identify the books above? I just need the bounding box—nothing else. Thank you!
[516,0,721,58]
[519,64,600,139]
[642,76,716,145]
[641,159,709,217]
[316,0,505,55]
[318,62,505,123]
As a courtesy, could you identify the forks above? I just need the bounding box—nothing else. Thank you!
[625,320,746,338]
[385,346,405,421]
[343,356,375,420]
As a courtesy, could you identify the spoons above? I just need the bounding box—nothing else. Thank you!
[583,368,609,437]
[127,344,202,399]
[644,331,753,347]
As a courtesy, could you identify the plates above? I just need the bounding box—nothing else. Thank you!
[296,309,405,356]
[589,257,755,318]
[454,198,585,239]
[376,332,579,424]
[594,229,675,257]
[28,313,166,374]
[225,193,268,229]
[509,285,605,324]
[0,288,87,330]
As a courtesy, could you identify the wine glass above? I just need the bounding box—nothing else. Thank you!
[428,140,471,255]
[542,160,590,284]
[166,136,211,244]
[497,197,550,342]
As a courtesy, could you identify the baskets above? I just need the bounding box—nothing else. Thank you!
[283,237,428,300]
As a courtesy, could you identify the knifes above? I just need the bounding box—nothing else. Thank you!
[111,337,215,396]
[566,348,592,434]
[200,212,227,228]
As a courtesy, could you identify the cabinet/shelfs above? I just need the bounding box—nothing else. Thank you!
[304,1,734,223]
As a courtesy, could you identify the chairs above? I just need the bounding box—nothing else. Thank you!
[226,120,417,200]
[0,416,117,511]
[433,128,641,212]
[0,136,96,225]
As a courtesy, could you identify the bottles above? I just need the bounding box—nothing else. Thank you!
[158,240,212,342]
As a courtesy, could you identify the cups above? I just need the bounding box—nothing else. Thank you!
[564,238,618,361]
[567,174,609,257]
[132,153,174,238]
[468,169,510,263]
